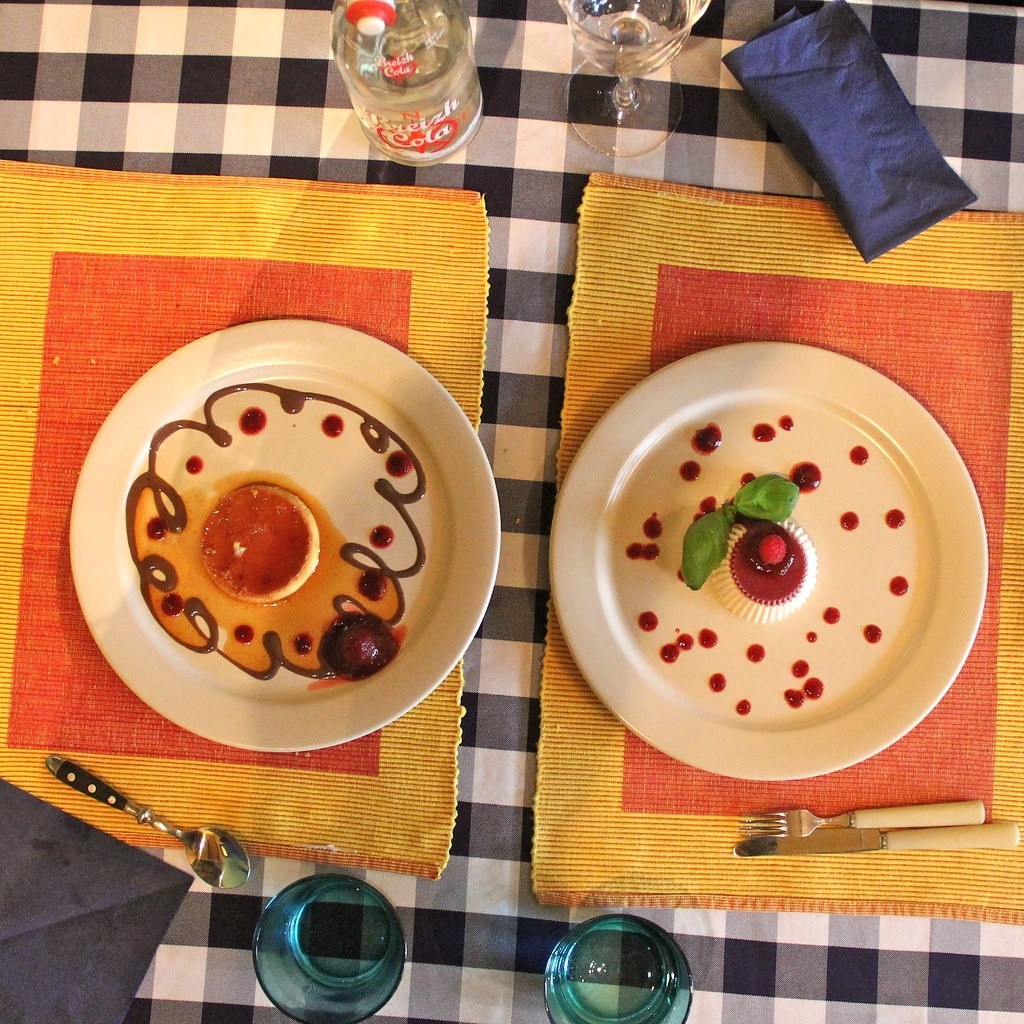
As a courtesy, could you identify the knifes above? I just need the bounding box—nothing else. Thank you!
[733,824,1021,856]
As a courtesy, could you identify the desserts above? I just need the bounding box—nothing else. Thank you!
[201,484,319,604]
[710,520,818,625]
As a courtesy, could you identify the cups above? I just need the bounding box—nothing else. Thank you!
[249,872,406,1024]
[543,913,694,1024]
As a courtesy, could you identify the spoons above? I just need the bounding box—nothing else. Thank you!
[46,754,250,890]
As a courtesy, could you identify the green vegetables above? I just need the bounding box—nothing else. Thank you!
[682,475,800,591]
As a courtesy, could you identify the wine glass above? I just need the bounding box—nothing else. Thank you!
[557,1,712,158]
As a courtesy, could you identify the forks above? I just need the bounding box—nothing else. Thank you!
[743,800,985,838]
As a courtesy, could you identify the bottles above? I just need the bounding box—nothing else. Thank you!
[332,0,485,168]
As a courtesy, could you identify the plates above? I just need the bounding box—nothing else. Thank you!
[70,320,506,753]
[548,342,988,783]
